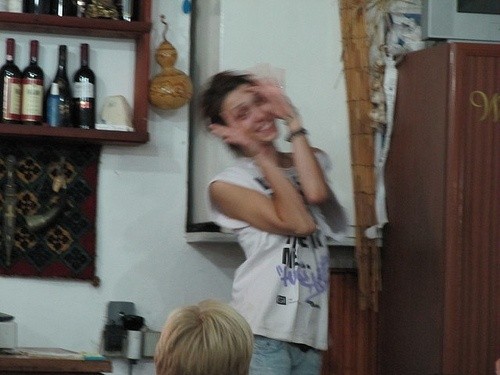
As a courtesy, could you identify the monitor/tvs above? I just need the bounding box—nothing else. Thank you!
[419,0,500,41]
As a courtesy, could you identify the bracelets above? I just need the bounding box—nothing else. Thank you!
[284,127,307,142]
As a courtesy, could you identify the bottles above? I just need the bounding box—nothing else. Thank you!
[43,45,74,126]
[20,40,45,125]
[0,37,22,125]
[71,43,95,129]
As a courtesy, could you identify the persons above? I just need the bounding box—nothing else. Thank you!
[201,72,331,375]
[155,298,254,375]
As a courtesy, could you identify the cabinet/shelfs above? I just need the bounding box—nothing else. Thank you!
[0,0,152,142]
[378,41,500,375]
